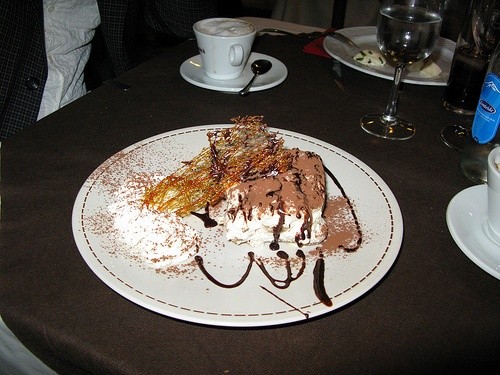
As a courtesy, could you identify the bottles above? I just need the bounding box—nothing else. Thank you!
[456,43,500,185]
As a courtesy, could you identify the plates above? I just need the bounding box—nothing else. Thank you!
[323,26,457,86]
[446,183,500,280]
[71,124,404,329]
[179,51,289,95]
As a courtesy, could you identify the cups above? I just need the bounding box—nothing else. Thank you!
[192,17,258,80]
[442,0,500,116]
[486,146,500,247]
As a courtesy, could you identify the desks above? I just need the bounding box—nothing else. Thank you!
[1,17,500,374]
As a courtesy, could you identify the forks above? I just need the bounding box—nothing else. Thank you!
[259,28,360,48]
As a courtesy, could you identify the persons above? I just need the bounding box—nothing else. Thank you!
[0,0,217,141]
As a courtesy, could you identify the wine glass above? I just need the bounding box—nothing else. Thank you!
[359,0,448,140]
[439,120,473,153]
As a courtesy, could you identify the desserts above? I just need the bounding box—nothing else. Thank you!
[108,115,327,268]
[350,48,442,78]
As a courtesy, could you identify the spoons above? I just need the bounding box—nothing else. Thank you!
[239,59,272,94]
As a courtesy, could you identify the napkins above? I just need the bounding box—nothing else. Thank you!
[303,28,335,59]
